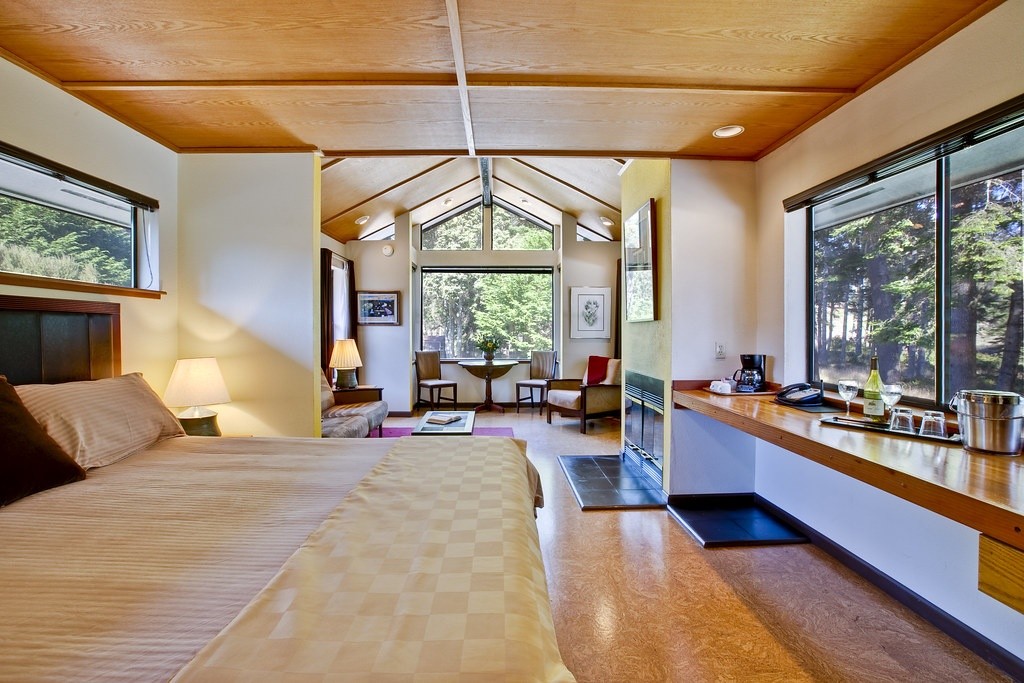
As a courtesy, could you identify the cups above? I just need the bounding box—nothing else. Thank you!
[925,411,948,438]
[919,416,945,439]
[889,407,912,414]
[710,381,722,390]
[889,412,917,434]
[717,383,732,393]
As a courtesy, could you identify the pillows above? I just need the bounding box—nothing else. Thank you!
[11,370,186,475]
[1,375,87,510]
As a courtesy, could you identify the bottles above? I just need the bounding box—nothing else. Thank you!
[863,356,885,424]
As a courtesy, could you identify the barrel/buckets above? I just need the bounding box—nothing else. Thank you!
[949,390,1023,454]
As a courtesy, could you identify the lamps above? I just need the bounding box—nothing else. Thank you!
[328,337,363,389]
[162,356,231,438]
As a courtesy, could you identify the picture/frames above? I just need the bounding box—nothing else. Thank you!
[356,291,402,328]
[623,198,658,323]
[568,286,615,340]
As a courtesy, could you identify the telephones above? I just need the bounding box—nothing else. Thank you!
[777,383,821,403]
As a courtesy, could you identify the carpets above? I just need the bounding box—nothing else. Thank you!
[368,426,514,438]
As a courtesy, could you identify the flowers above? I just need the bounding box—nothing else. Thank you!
[0,295,576,683]
[476,334,500,352]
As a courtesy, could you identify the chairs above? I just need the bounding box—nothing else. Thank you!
[413,350,458,412]
[546,355,622,433]
[320,367,389,439]
[514,351,557,416]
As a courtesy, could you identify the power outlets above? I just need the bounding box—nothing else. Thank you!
[717,342,726,359]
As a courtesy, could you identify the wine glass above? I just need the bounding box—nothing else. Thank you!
[878,385,903,424]
[838,379,859,419]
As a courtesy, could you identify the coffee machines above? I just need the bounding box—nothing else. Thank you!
[733,354,766,390]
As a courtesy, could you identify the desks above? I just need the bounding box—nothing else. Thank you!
[332,385,384,439]
[411,409,477,436]
[458,362,519,413]
[671,388,1024,614]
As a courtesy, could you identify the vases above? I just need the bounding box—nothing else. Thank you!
[484,350,495,365]
